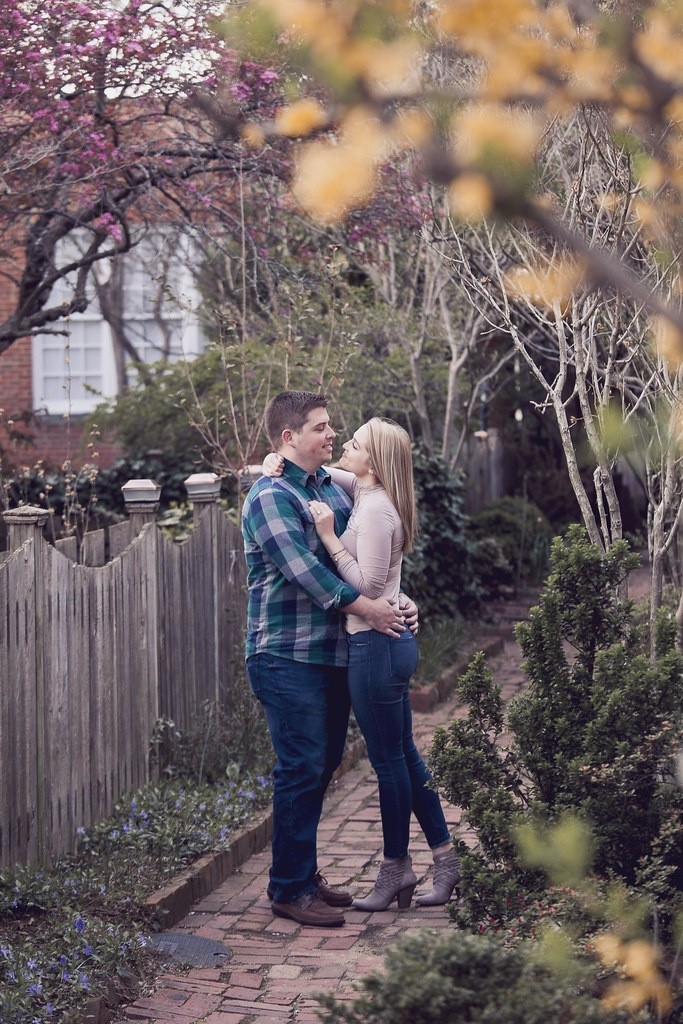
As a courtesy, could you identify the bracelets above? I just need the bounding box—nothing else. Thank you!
[330,548,348,563]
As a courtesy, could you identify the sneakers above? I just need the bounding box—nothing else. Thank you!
[267,870,354,925]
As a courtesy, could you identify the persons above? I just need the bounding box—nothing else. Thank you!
[239,391,419,925]
[263,418,462,911]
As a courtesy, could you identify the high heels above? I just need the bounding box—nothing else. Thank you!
[352,855,417,911]
[415,846,463,904]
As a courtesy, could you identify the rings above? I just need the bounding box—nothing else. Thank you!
[317,511,322,515]
